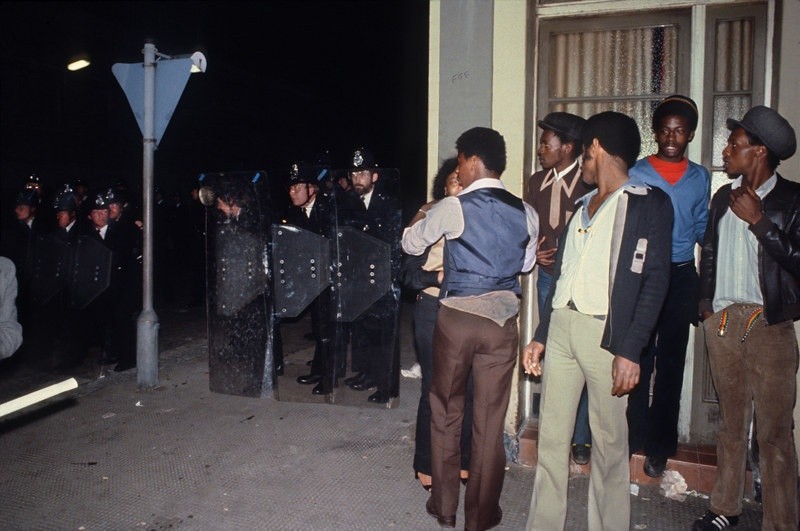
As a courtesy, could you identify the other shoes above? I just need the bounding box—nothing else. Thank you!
[414,471,433,492]
[644,455,668,477]
[344,371,378,391]
[368,390,399,403]
[571,442,592,465]
[460,477,469,486]
[306,360,314,366]
[464,506,502,531]
[426,496,457,528]
[275,364,284,376]
[312,380,339,395]
[303,333,312,341]
[296,373,322,384]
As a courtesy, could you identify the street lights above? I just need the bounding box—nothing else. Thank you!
[111,43,208,389]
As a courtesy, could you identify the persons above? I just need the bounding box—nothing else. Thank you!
[0,144,403,403]
[525,113,598,463]
[400,126,540,531]
[693,107,800,531]
[404,158,487,490]
[629,95,709,476]
[520,111,675,531]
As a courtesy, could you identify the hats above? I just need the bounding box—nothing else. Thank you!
[17,173,126,212]
[651,95,698,132]
[289,161,323,188]
[726,106,797,160]
[347,146,379,172]
[215,181,237,201]
[539,112,587,142]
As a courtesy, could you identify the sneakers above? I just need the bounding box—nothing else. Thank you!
[691,509,740,531]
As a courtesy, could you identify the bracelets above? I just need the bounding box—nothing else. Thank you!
[418,208,426,214]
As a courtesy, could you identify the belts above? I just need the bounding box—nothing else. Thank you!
[568,302,606,321]
